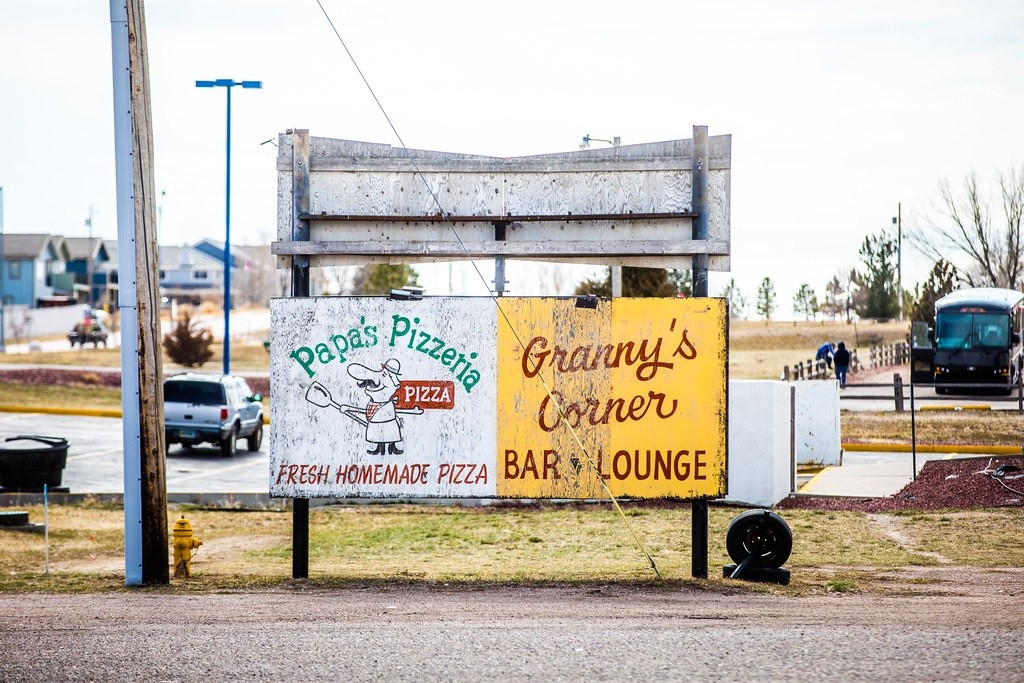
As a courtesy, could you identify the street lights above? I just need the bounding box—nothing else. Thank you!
[578,134,624,298]
[194,79,263,375]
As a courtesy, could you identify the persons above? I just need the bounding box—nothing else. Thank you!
[816,342,835,370]
[834,342,849,392]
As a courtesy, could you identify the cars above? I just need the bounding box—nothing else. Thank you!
[162,371,266,457]
[69,319,107,348]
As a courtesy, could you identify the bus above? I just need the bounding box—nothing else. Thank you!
[910,287,1022,396]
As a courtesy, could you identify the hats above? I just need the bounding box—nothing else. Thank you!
[831,343,835,350]
